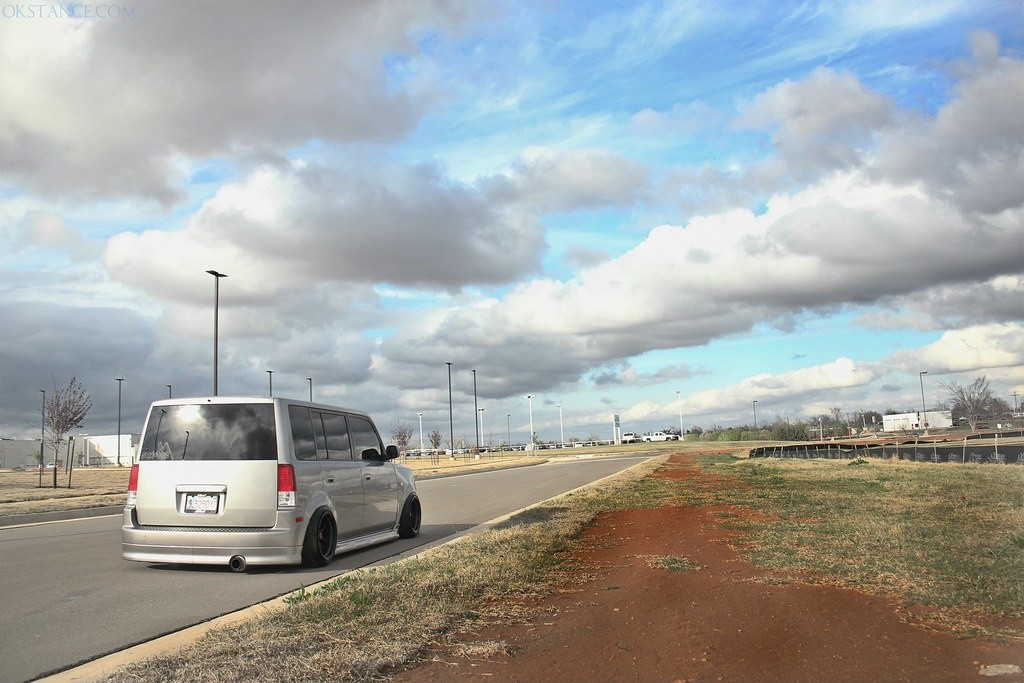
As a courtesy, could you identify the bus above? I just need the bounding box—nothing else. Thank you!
[622,432,636,443]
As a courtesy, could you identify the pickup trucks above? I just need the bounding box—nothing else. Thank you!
[642,432,679,442]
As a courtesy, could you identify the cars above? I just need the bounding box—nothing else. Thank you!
[404,441,602,456]
[954,412,1024,421]
[46,463,58,468]
[876,421,884,432]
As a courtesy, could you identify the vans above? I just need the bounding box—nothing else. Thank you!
[119,395,421,572]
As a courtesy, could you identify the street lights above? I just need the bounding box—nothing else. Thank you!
[446,362,454,457]
[472,370,479,454]
[557,404,563,445]
[39,390,45,473]
[479,408,484,447]
[267,371,275,397]
[307,378,312,402]
[154,408,167,458]
[523,396,535,450]
[207,269,228,395]
[417,413,424,452]
[182,431,189,459]
[116,378,125,465]
[508,415,511,445]
[920,372,928,431]
[753,400,757,428]
[847,414,851,438]
[676,390,683,439]
[167,385,171,398]
